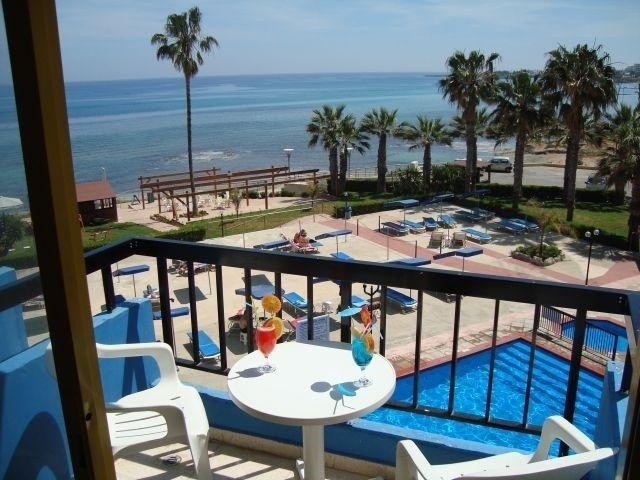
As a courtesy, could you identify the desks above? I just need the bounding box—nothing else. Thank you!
[227,339,397,480]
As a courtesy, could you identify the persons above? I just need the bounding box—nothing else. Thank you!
[294,229,304,242]
[239,310,257,345]
[146,284,160,300]
[78,214,85,229]
[299,232,312,247]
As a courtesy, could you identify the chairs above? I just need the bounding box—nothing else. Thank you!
[395,412,620,478]
[47,333,210,480]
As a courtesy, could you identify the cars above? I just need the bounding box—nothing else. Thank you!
[484,157,513,173]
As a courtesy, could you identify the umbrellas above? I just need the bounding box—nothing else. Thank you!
[460,189,492,216]
[389,199,419,227]
[0,195,24,229]
[112,264,150,298]
[431,194,454,221]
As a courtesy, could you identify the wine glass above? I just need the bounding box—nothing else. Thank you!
[237,367,262,380]
[351,336,375,387]
[333,381,359,397]
[255,326,277,373]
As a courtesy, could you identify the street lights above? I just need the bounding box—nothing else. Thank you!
[582,228,600,285]
[343,146,356,179]
[282,146,296,178]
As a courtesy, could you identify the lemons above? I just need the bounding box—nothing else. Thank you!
[263,316,284,341]
[364,333,375,354]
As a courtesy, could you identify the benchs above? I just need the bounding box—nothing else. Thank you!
[186,330,221,365]
[283,251,418,322]
[381,209,540,248]
